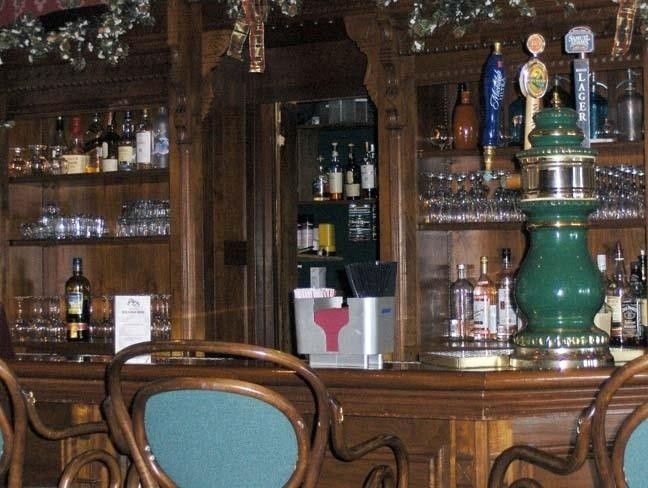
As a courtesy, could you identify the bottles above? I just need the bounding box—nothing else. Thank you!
[49,106,170,174]
[449,67,645,151]
[310,140,380,204]
[296,220,337,257]
[64,255,92,342]
[448,240,648,346]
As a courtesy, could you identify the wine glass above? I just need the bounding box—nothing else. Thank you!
[10,291,172,345]
[421,162,646,224]
[20,197,170,239]
[7,142,67,179]
